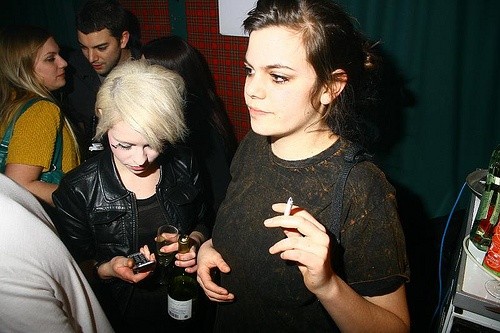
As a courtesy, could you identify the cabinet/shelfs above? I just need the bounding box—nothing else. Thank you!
[439,168,500,333]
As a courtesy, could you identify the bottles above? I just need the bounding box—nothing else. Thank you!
[86,116,104,161]
[166,233,198,333]
[470,149,500,251]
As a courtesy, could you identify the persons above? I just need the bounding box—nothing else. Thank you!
[52,59,214,333]
[141,36,237,208]
[196,0,409,333]
[0,28,80,209]
[56,7,141,156]
[0,173,115,332]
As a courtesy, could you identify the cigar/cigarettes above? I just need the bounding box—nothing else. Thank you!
[284,197,293,215]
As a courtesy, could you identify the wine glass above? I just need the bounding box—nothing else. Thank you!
[485,280,500,300]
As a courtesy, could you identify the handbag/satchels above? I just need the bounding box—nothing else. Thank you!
[0,96,67,214]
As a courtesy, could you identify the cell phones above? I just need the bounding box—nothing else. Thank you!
[127,253,155,273]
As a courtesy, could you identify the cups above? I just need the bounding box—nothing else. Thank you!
[156,225,178,266]
